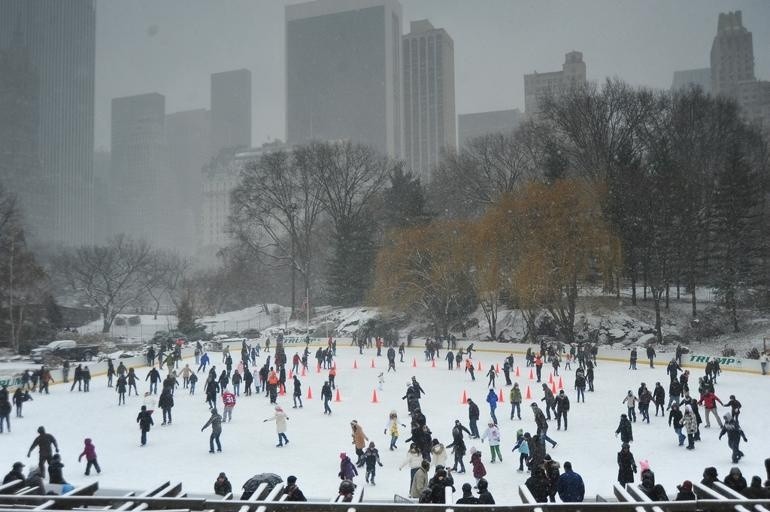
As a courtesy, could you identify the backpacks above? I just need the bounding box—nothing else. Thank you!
[419,485,434,503]
[410,467,421,491]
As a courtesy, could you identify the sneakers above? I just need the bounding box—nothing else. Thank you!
[389,443,397,450]
[630,413,725,430]
[510,414,523,421]
[552,442,557,448]
[276,440,289,447]
[292,404,303,409]
[209,448,222,453]
[323,410,332,416]
[184,385,287,397]
[161,421,172,426]
[546,417,569,432]
[365,477,376,485]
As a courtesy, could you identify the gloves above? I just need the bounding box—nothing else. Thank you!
[384,428,387,435]
[378,463,383,467]
[541,397,546,402]
[401,423,407,427]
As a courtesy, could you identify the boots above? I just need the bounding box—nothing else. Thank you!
[458,466,465,473]
[450,463,457,471]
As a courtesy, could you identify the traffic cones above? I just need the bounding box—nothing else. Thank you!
[277,354,565,405]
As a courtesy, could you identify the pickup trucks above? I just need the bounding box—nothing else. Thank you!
[29,339,99,365]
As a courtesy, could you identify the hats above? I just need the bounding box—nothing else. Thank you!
[422,459,429,468]
[369,442,375,448]
[640,369,735,410]
[544,453,551,460]
[340,451,346,459]
[470,446,477,454]
[488,417,494,424]
[524,432,530,438]
[514,382,518,388]
[462,483,471,492]
[621,442,631,448]
[12,461,25,467]
[530,402,537,407]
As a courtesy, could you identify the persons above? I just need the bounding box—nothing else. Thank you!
[0,324,769,504]
